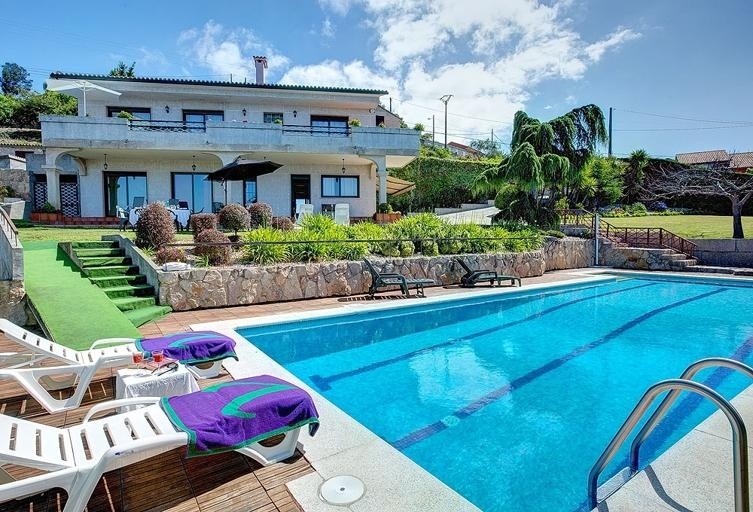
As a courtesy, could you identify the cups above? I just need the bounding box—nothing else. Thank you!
[143,351,149,359]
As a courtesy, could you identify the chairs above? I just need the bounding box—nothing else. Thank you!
[452,255,521,288]
[0,372,321,512]
[0,314,238,416]
[114,195,222,232]
[361,255,436,299]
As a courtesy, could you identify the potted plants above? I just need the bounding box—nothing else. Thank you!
[30,200,64,221]
[373,202,401,223]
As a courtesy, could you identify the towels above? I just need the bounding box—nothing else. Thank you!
[160,374,321,461]
[133,329,238,365]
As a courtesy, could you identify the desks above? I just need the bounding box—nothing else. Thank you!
[321,203,350,226]
[110,362,201,415]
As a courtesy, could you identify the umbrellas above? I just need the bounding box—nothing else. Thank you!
[202,156,284,207]
[46,78,122,116]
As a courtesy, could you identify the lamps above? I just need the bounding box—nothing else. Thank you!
[164,104,171,114]
[292,110,298,118]
[340,158,346,174]
[102,153,109,171]
[191,155,197,171]
[241,108,246,116]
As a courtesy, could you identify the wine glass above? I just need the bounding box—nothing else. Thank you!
[151,350,163,374]
[132,351,143,376]
